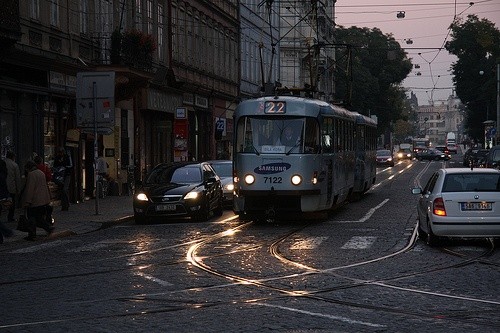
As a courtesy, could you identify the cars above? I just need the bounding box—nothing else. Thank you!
[397,148,412,160]
[417,148,451,162]
[464,145,500,170]
[447,143,457,154]
[132,161,224,223]
[376,150,394,167]
[209,160,233,210]
[411,167,500,248]
[435,146,449,154]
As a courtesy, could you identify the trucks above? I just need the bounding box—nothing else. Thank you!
[413,138,430,159]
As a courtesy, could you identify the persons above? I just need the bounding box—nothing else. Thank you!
[96,150,108,195]
[277,124,301,147]
[0,148,73,242]
[24,160,56,241]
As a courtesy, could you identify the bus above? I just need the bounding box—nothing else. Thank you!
[231,94,378,221]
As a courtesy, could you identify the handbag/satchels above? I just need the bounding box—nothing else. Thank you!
[16,207,32,232]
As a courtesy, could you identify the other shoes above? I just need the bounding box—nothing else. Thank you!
[8,219,16,222]
[24,237,36,242]
